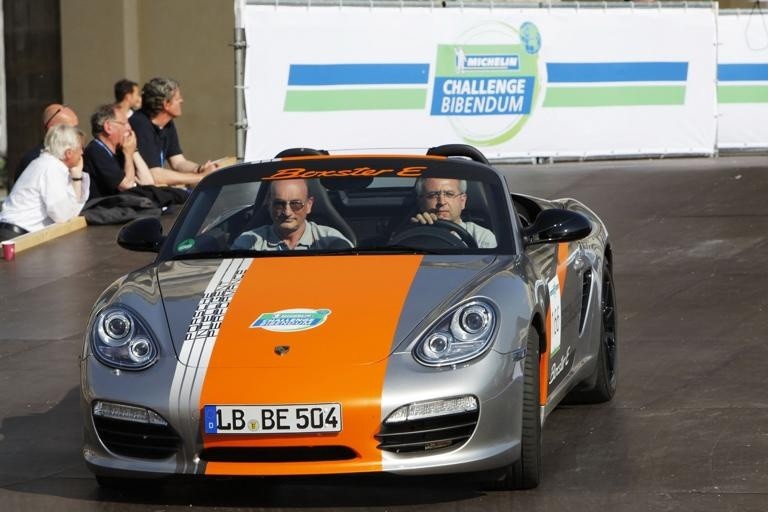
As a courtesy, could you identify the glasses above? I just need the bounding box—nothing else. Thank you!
[419,190,464,200]
[271,198,309,212]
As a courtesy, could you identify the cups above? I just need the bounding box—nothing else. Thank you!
[0,241,16,261]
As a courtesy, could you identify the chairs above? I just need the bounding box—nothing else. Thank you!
[389,143,501,236]
[241,148,358,249]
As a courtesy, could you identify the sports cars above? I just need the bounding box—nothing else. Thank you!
[77,143,620,492]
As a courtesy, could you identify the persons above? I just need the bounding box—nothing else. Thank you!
[411,178,498,249]
[0,76,220,244]
[229,179,355,251]
